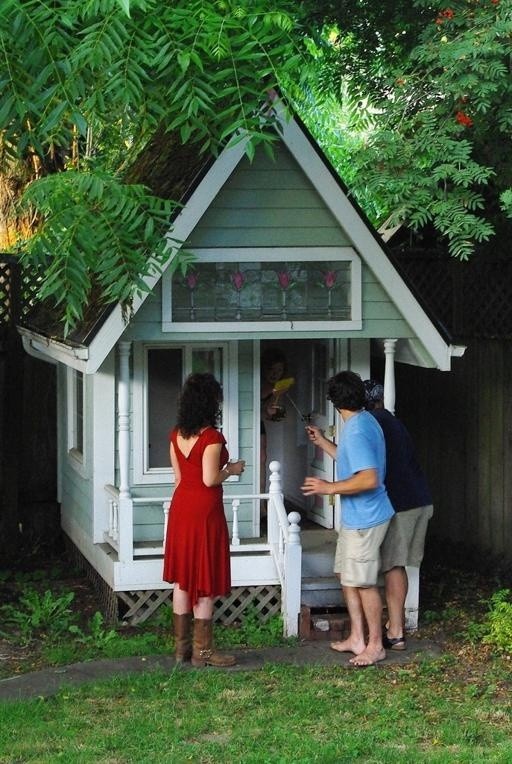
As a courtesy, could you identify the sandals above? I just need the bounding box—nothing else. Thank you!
[366,626,407,650]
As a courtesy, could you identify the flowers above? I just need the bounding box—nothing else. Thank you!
[185,271,344,321]
[272,377,308,426]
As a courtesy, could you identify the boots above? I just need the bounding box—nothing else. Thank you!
[172,613,236,668]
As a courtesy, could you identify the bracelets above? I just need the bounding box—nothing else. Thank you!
[224,468,229,474]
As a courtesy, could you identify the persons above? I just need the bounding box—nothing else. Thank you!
[163,373,246,667]
[362,379,434,650]
[300,370,395,666]
